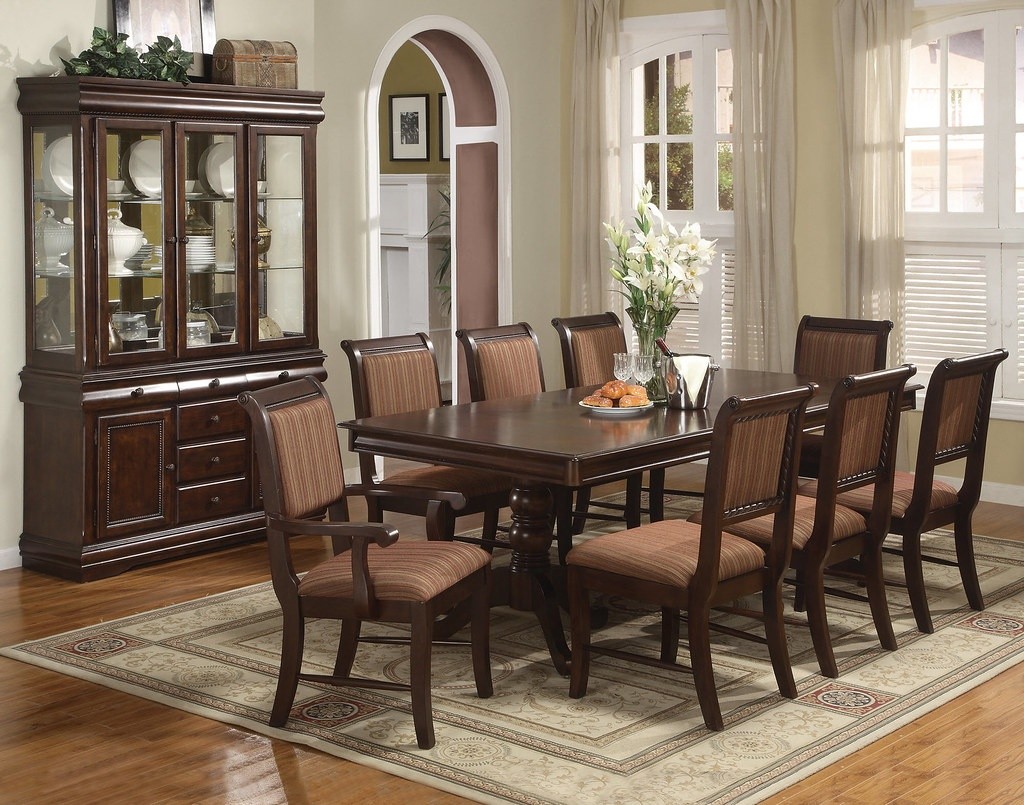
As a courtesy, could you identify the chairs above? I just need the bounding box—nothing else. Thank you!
[236,311,1009,750]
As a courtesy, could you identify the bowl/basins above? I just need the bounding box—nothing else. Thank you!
[185,181,195,193]
[257,181,268,193]
[33,179,45,192]
[107,179,125,194]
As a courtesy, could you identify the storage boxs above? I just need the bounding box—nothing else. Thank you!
[211,39,298,89]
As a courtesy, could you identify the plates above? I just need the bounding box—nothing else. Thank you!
[48,137,274,201]
[34,135,69,197]
[578,400,654,414]
[154,235,217,273]
[125,244,153,270]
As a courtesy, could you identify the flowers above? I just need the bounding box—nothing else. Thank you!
[603,177,719,311]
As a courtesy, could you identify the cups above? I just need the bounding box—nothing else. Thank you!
[612,352,634,382]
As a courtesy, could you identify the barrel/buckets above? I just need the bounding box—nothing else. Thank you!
[658,354,720,410]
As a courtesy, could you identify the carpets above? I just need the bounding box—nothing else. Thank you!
[0,486,1024,805]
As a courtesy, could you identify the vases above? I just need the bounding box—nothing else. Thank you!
[625,305,680,400]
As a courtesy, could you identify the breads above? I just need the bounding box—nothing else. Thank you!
[583,379,650,408]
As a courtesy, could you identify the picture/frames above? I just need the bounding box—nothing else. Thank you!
[388,93,430,162]
[112,0,216,84]
[437,92,450,161]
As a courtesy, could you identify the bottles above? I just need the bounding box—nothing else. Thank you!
[110,311,149,340]
[655,337,676,357]
[158,319,211,348]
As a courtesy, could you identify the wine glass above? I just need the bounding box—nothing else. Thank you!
[634,355,654,388]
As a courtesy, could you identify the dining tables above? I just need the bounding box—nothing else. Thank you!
[334,368,925,677]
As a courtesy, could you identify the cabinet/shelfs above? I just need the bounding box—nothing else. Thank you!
[16,76,328,584]
